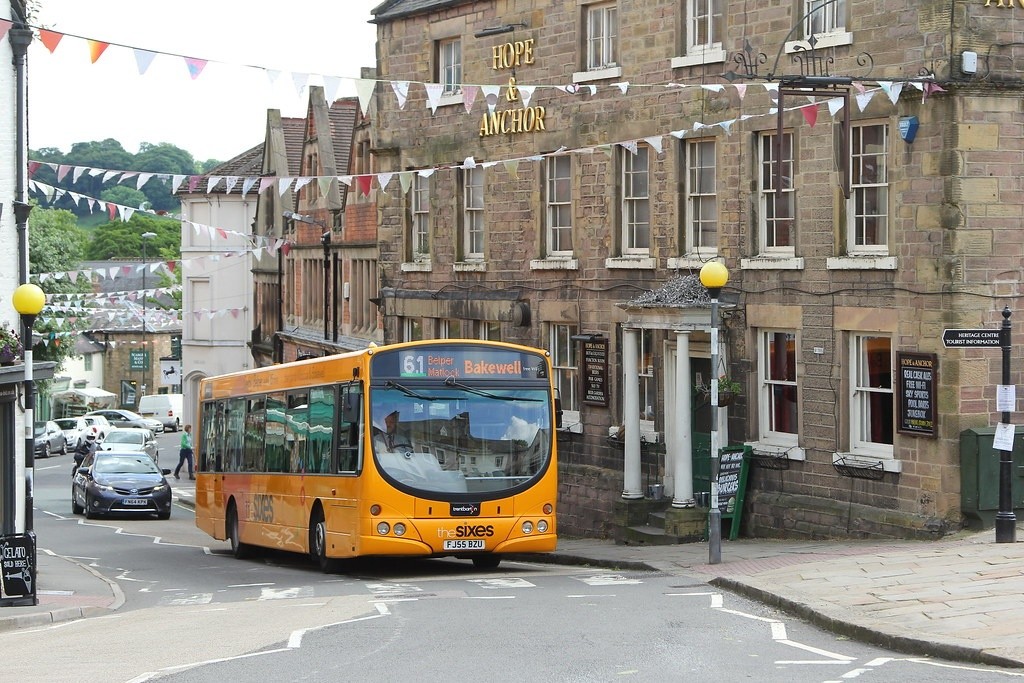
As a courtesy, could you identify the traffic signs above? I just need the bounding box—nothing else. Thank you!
[941,328,1001,349]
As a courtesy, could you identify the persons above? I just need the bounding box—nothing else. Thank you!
[173,425,196,480]
[373,406,412,454]
[77,465,93,474]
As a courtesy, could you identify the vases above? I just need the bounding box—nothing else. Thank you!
[709,391,734,407]
[0,355,16,364]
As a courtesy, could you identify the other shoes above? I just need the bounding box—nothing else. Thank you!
[190,476,196,480]
[174,473,180,479]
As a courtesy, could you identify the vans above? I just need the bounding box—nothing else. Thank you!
[137,394,183,433]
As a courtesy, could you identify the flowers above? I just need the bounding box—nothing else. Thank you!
[693,375,743,401]
[0,326,24,358]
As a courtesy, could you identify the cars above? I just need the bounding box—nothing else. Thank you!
[81,408,164,437]
[33,420,68,458]
[96,427,160,466]
[72,450,172,520]
[50,416,90,453]
[72,414,116,466]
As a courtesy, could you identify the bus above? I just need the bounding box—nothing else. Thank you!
[195,338,562,575]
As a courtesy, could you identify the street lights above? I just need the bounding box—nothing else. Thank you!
[141,231,158,395]
[12,283,45,533]
[699,261,729,565]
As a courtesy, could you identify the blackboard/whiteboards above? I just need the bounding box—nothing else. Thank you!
[718,446,752,518]
[580,338,609,407]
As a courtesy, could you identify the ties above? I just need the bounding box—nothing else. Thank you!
[388,436,395,453]
